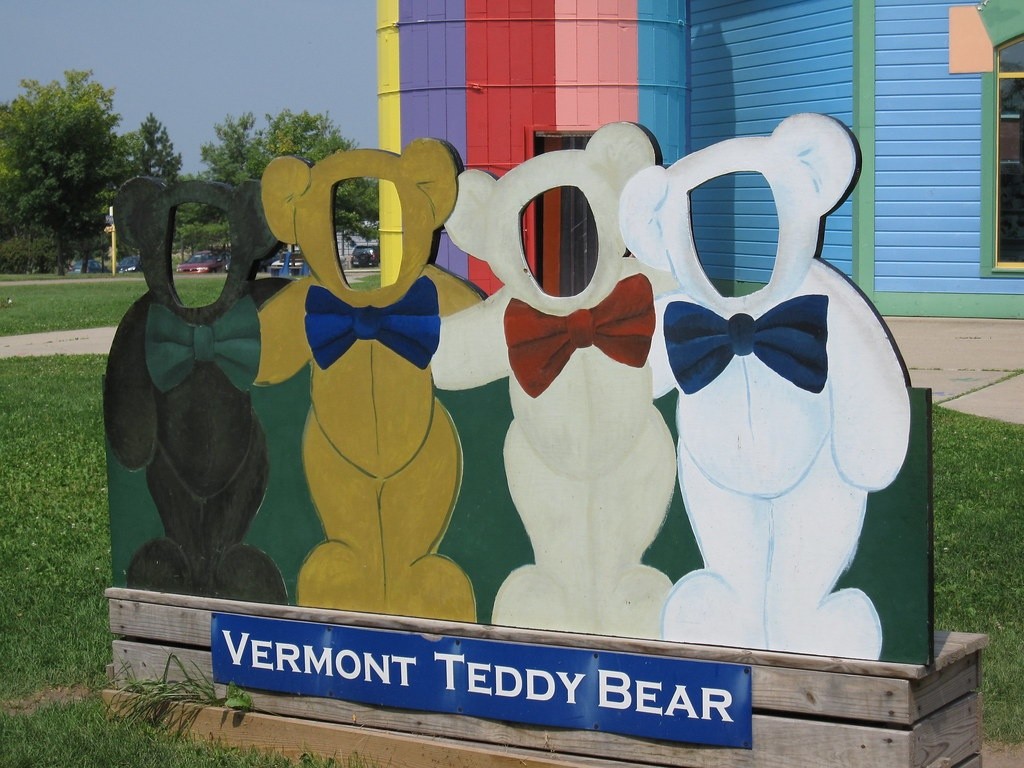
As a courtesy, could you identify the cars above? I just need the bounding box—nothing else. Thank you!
[176,250,223,273]
[67,260,111,274]
[116,257,142,273]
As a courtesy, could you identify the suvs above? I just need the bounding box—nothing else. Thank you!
[350,245,380,268]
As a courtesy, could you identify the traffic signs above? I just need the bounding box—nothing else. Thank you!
[103,226,116,233]
